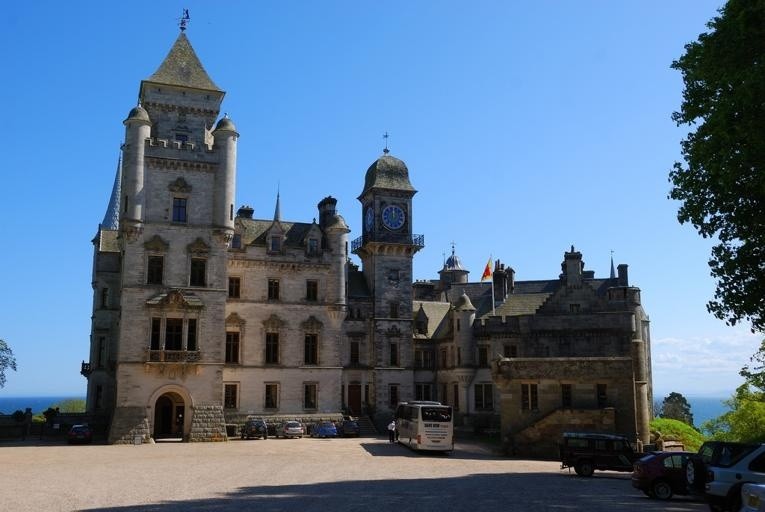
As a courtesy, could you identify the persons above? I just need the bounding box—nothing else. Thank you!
[653,431,665,451]
[176,414,184,438]
[388,421,396,445]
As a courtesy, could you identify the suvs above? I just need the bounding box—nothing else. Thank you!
[557,431,655,479]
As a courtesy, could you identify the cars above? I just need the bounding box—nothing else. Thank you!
[685,438,765,512]
[67,423,93,444]
[629,448,702,500]
[239,415,363,440]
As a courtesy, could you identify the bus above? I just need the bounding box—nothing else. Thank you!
[394,398,458,453]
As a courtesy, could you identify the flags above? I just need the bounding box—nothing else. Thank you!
[480,255,492,282]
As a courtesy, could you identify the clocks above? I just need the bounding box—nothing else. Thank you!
[361,205,374,233]
[381,202,408,232]
